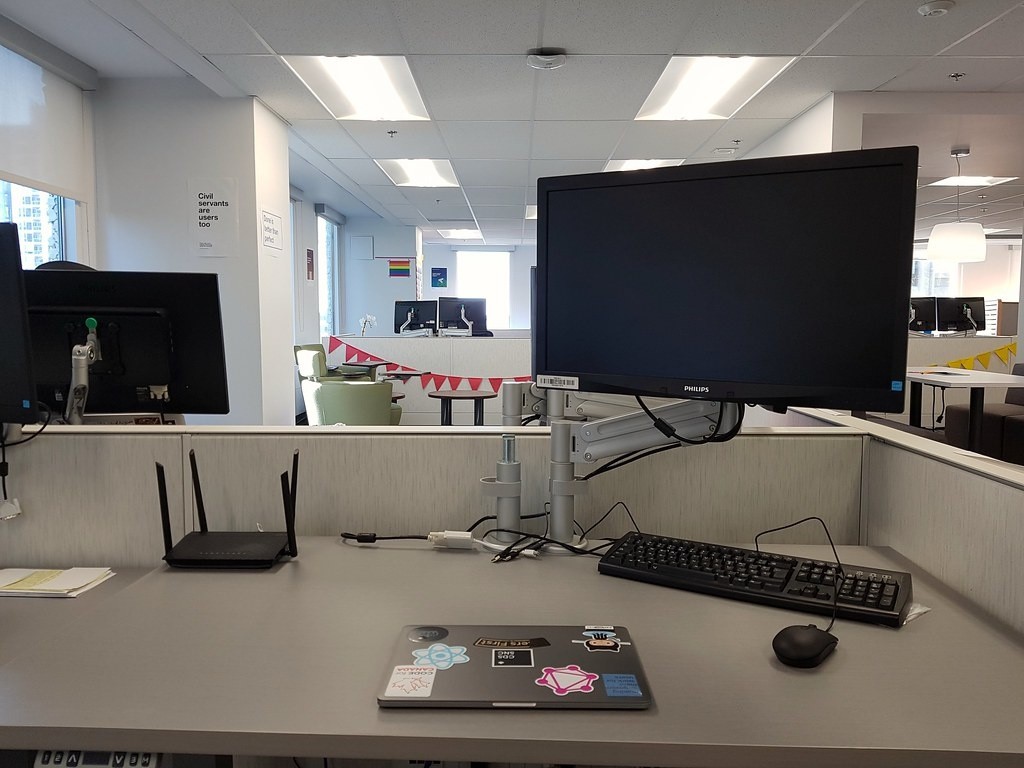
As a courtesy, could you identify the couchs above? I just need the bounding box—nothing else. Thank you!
[294,343,402,425]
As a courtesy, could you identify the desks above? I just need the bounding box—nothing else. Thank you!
[341,361,392,382]
[0,534,1024,768]
[378,371,431,382]
[391,392,405,404]
[428,390,498,426]
[906,366,1024,453]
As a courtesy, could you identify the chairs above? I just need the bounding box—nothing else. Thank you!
[947,363,1024,466]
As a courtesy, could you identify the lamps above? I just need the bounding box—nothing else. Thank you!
[926,148,987,263]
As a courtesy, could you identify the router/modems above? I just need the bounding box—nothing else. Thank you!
[155,449,300,570]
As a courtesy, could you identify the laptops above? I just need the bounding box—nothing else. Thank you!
[377,624,651,711]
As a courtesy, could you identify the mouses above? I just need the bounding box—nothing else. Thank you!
[772,623,839,669]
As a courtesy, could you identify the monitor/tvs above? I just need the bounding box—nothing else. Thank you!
[0,221,29,425]
[394,301,437,332]
[22,268,230,418]
[438,297,487,331]
[936,297,986,332]
[536,144,919,413]
[908,296,936,331]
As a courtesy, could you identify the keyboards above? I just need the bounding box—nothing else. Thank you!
[598,530,913,630]
